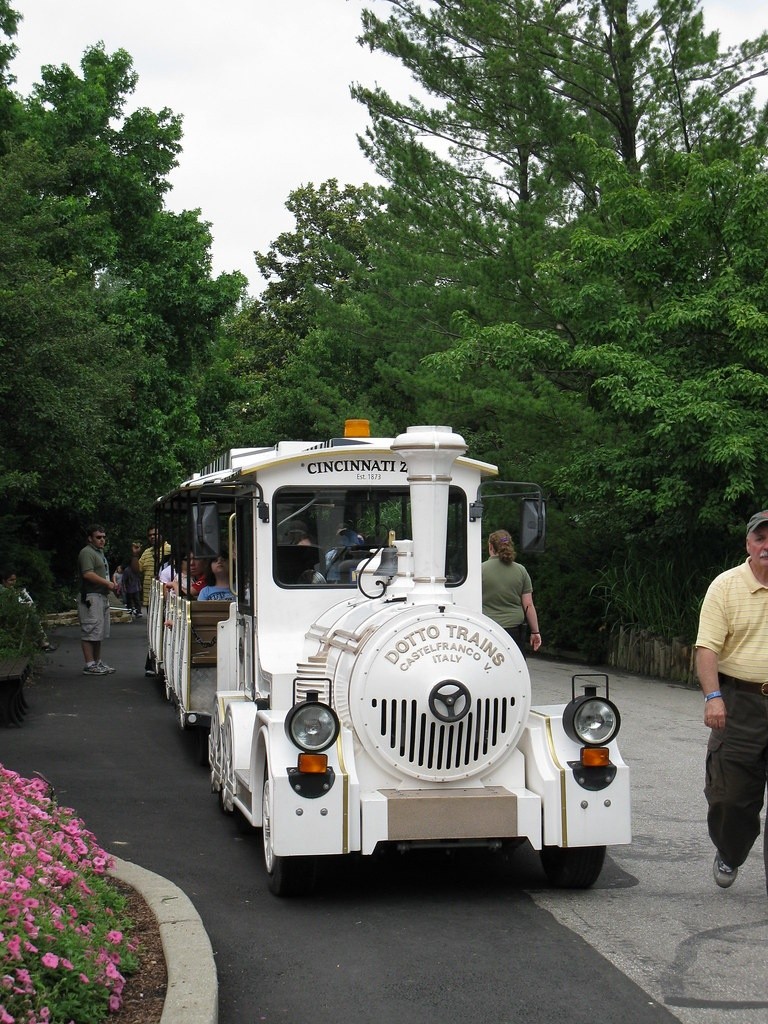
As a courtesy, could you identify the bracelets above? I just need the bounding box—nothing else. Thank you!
[705,691,722,702]
[531,632,540,634]
[133,555,140,557]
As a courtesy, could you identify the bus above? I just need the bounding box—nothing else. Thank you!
[148,418,630,897]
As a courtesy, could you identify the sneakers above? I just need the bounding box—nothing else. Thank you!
[712,849,738,888]
[97,661,116,674]
[82,664,107,675]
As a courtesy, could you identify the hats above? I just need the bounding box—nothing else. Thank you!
[283,520,308,535]
[335,522,349,536]
[746,509,768,533]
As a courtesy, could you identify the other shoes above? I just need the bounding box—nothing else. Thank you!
[123,601,143,618]
[41,645,58,653]
[145,669,156,678]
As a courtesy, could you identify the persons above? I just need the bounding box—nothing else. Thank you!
[131,526,172,677]
[286,520,313,545]
[122,561,143,617]
[113,565,127,607]
[693,510,768,895]
[75,524,116,675]
[159,540,237,631]
[325,523,365,571]
[0,570,58,652]
[481,530,542,662]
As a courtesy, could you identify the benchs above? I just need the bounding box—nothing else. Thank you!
[189,599,233,664]
[0,656,32,729]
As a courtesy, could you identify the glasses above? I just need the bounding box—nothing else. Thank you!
[96,535,106,539]
